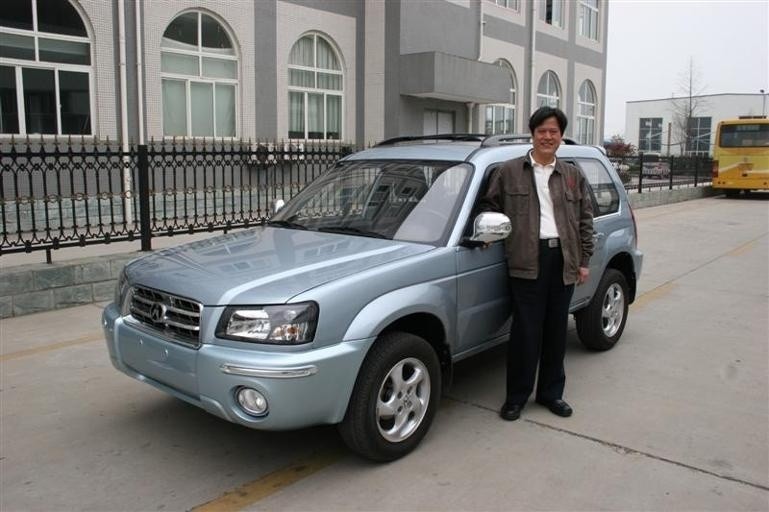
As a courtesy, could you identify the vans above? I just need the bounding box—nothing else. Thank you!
[641,152,671,179]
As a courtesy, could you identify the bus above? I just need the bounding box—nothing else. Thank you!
[711,114,769,198]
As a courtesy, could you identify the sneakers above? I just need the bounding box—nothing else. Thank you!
[499,399,526,421]
[534,398,573,419]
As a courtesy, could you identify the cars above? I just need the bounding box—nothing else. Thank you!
[608,158,630,172]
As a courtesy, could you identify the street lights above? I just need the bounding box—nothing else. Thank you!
[760,88,766,118]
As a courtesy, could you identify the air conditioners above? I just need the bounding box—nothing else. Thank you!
[247,142,305,167]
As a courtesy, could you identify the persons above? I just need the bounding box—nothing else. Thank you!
[476,106,595,421]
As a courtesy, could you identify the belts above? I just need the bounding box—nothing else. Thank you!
[538,238,561,250]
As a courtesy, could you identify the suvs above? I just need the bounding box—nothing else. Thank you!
[101,131,645,465]
